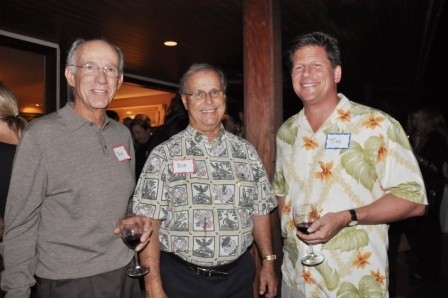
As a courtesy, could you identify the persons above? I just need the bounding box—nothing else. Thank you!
[0,38,153,298]
[0,83,29,240]
[130,64,279,298]
[405,105,448,282]
[106,101,246,181]
[271,32,429,298]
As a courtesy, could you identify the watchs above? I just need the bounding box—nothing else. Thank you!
[347,209,357,227]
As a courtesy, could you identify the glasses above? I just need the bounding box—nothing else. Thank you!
[75,63,121,77]
[186,89,224,100]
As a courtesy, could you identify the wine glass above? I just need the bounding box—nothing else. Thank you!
[119,213,151,278]
[293,202,325,266]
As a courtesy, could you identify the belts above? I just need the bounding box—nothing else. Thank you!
[194,267,229,276]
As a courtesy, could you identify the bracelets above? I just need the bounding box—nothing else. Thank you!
[421,157,424,164]
[262,254,277,261]
